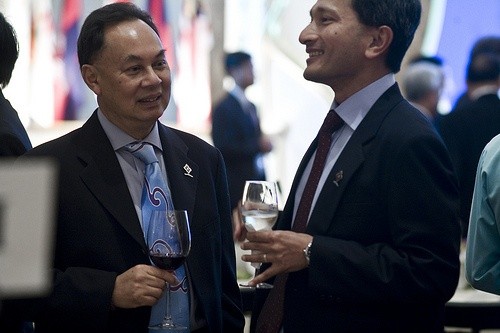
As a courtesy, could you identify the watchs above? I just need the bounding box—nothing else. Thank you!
[303,241,312,262]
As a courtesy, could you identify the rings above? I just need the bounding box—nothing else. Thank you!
[263,253,268,262]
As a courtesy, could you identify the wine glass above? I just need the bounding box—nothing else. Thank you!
[147,209,191,330]
[238,181,279,289]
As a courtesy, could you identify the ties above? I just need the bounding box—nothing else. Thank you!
[252,110,346,333]
[125,140,194,333]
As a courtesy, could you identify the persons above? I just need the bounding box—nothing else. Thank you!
[0,12,33,164]
[237,0,462,332]
[212,51,275,206]
[466,133,500,295]
[8,1,248,333]
[399,35,500,240]
